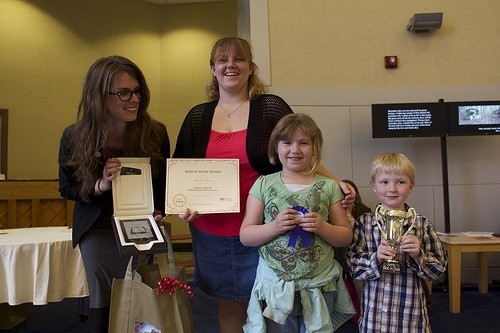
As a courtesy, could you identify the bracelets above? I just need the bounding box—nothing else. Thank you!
[98,178,105,193]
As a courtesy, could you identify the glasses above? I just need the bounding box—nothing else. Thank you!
[109,87,143,102]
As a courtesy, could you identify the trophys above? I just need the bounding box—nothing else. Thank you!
[375,205,417,274]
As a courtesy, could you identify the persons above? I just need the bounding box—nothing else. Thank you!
[341,179,371,221]
[58,55,170,333]
[239,112,353,333]
[344,151,449,333]
[173,36,355,333]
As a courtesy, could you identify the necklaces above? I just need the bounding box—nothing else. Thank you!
[218,99,248,118]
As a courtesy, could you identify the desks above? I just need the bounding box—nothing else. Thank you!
[0,225,90,333]
[424,231,500,314]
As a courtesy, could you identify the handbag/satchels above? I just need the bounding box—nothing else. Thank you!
[109,224,194,333]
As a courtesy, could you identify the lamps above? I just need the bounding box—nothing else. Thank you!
[405,12,443,34]
